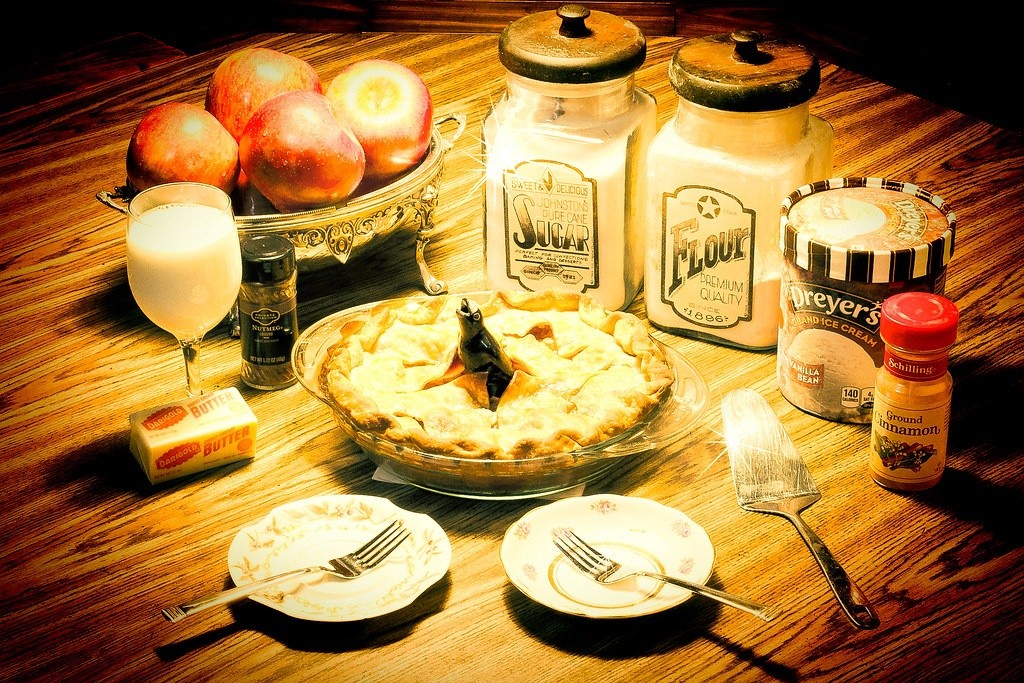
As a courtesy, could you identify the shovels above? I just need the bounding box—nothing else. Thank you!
[720,388,881,630]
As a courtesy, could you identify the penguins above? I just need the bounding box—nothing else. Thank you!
[455,297,515,398]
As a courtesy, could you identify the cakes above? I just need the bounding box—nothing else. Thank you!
[320,284,676,492]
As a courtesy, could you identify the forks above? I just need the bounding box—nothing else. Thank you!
[552,524,784,623]
[159,518,412,624]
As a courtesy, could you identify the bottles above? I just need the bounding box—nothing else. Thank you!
[239,233,302,392]
[866,291,961,493]
[777,175,956,425]
[643,29,836,352]
[482,4,656,311]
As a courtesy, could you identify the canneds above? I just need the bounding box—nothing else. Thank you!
[644,27,839,350]
[775,176,956,424]
[479,5,660,311]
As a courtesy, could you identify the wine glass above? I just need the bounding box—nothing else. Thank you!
[124,182,242,399]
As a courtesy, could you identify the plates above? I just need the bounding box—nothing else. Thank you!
[95,111,490,339]
[499,493,716,621]
[290,292,712,501]
[227,494,452,623]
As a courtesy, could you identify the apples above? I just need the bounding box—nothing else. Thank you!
[124,47,432,213]
